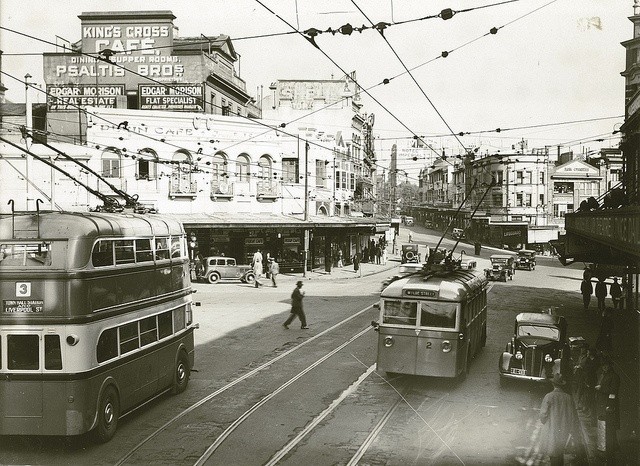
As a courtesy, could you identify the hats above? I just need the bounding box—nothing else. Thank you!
[550,373,566,386]
[296,281,304,285]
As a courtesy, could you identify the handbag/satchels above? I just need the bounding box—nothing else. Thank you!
[564,433,575,455]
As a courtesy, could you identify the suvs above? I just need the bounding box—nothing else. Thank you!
[196,257,255,284]
[484,254,515,282]
[400,244,420,263]
[515,249,536,270]
[498,307,568,384]
[452,227,465,240]
[405,217,414,226]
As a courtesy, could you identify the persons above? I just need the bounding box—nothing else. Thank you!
[253,249,263,266]
[408,233,413,243]
[611,277,623,309]
[582,277,593,309]
[577,342,625,451]
[266,253,271,279]
[253,258,264,288]
[597,279,608,311]
[364,235,392,265]
[281,280,310,330]
[268,257,280,287]
[537,371,576,466]
[352,254,360,273]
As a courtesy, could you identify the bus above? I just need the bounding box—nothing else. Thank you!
[0,204,201,442]
[371,259,487,379]
